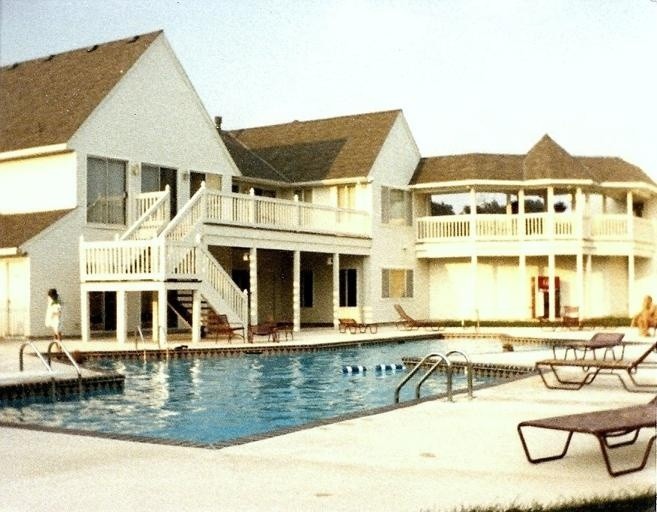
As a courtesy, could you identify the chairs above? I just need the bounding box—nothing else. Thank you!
[247,320,294,342]
[393,303,445,333]
[516,395,657,476]
[536,340,656,395]
[338,318,377,334]
[208,312,245,344]
[551,334,625,361]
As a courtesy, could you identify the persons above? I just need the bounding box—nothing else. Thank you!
[501,343,513,352]
[44,288,63,353]
[628,295,655,336]
